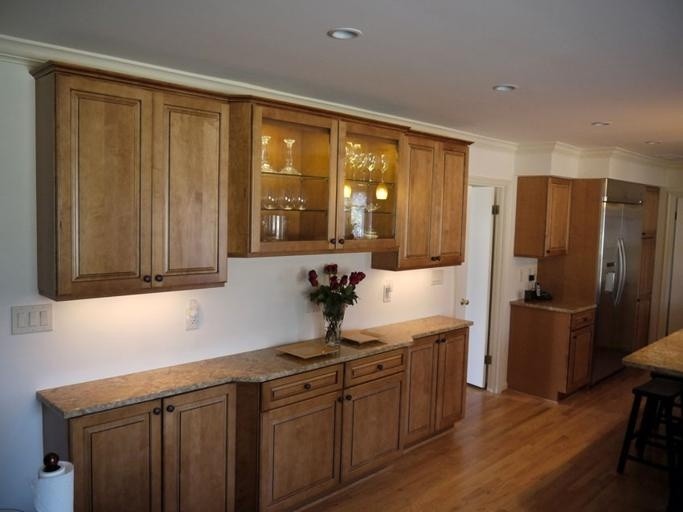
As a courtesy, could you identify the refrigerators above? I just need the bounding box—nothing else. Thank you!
[588,176,645,385]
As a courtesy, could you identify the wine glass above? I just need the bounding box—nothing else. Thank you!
[345,140,390,181]
[259,133,301,175]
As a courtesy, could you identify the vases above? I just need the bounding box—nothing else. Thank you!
[323,310,344,345]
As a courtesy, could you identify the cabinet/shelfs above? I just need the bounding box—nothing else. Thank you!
[42,385,238,512]
[406,327,469,449]
[514,175,573,258]
[507,299,598,401]
[236,346,411,512]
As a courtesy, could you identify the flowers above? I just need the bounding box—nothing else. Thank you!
[308,263,366,342]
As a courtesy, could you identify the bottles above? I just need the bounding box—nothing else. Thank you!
[528,274,540,298]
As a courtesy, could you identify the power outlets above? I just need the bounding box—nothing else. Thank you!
[529,268,538,281]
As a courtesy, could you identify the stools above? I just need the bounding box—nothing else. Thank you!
[617,377,683,486]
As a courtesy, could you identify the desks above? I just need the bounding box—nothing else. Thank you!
[621,326,683,449]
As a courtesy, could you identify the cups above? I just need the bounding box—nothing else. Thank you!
[262,215,286,240]
[260,189,310,210]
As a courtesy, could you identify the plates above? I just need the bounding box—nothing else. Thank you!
[275,341,337,360]
[340,328,382,346]
